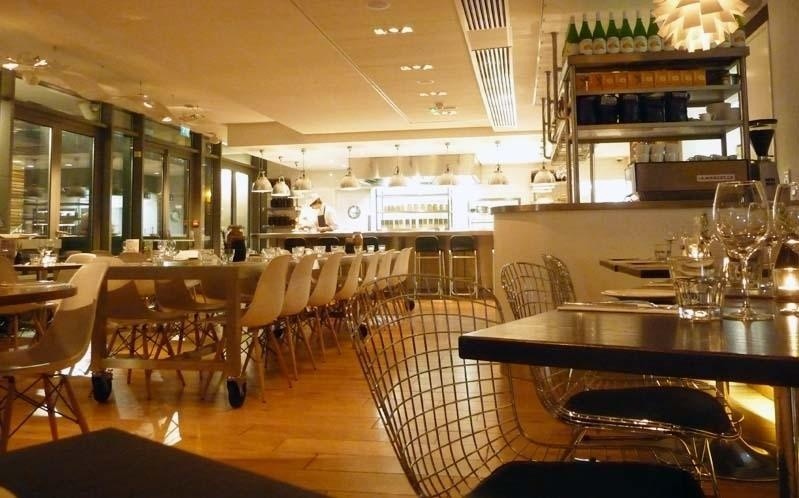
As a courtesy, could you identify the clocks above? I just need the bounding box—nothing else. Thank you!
[348,205,360,219]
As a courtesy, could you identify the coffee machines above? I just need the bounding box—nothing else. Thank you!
[744,117,781,186]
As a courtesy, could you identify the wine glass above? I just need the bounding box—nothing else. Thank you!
[772,184,799,266]
[27,239,57,263]
[711,181,775,321]
[748,201,784,291]
[157,240,176,258]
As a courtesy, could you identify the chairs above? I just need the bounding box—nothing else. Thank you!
[1,254,97,314]
[1,261,107,456]
[449,236,478,299]
[414,236,447,299]
[285,236,379,252]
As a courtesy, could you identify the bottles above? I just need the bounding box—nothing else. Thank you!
[605,11,621,53]
[619,11,633,53]
[593,12,606,54]
[647,10,665,53]
[632,10,649,53]
[578,14,593,53]
[565,15,580,55]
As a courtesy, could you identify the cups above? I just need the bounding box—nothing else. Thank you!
[144,240,153,261]
[699,112,715,120]
[262,245,387,257]
[653,245,669,261]
[772,268,799,296]
[665,236,727,321]
[199,249,215,264]
[219,248,236,264]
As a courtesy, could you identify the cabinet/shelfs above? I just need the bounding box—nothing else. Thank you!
[548,51,748,205]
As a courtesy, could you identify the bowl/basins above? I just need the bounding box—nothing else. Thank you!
[634,141,680,162]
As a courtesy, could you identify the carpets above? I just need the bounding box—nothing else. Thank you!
[0,428,332,498]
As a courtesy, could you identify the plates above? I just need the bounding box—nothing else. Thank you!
[602,287,677,302]
[708,101,740,121]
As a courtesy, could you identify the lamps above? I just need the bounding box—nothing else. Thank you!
[533,164,555,192]
[252,141,509,198]
[652,0,749,53]
